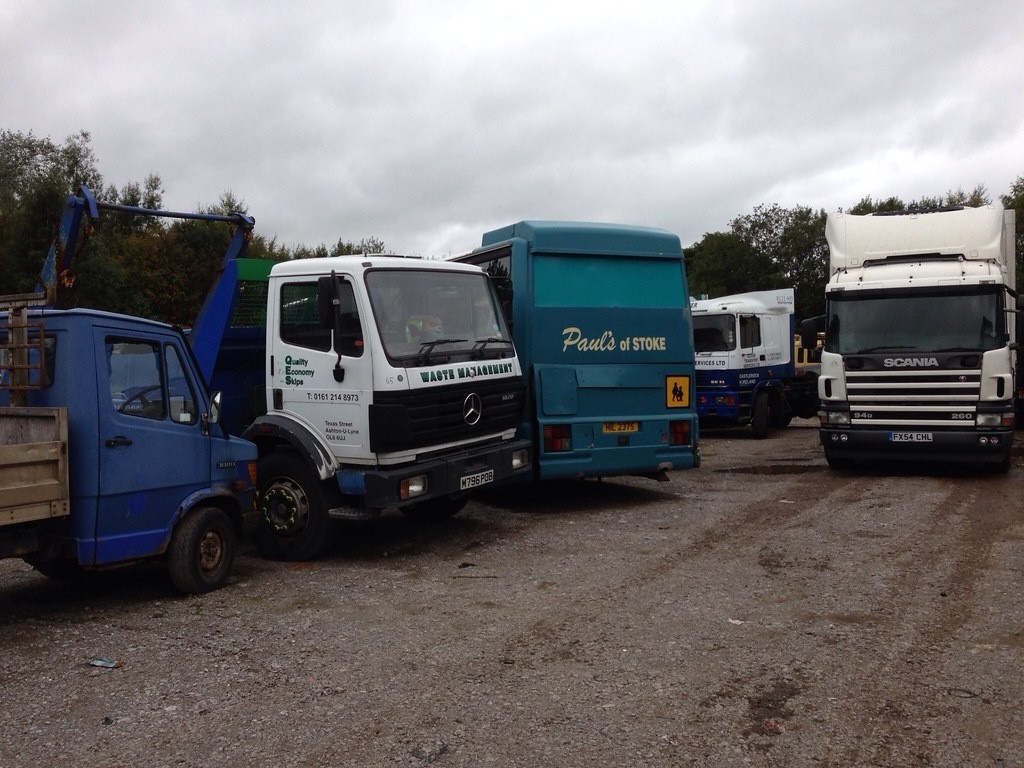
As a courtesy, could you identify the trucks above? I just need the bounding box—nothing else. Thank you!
[799,202,1022,474]
[0,305,265,593]
[692,286,827,440]
[168,252,536,561]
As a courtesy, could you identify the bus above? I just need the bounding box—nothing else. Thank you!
[453,223,701,497]
